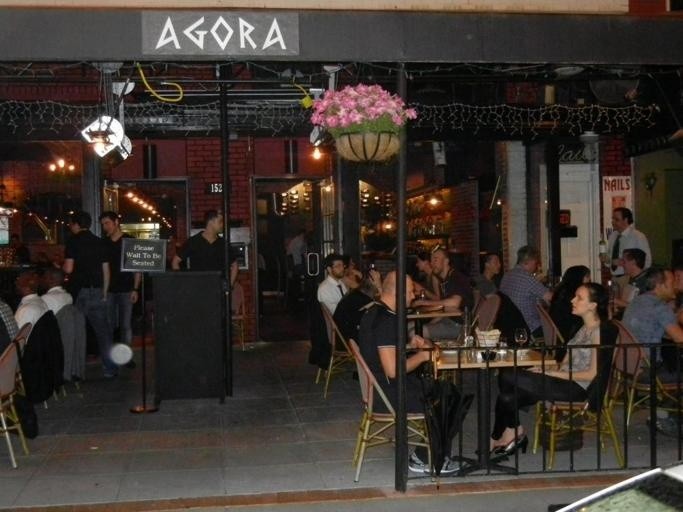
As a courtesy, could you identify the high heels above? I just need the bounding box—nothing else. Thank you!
[493,433,528,455]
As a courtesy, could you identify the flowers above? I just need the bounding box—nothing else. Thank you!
[310,82,417,139]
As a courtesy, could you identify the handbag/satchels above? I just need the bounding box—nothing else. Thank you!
[539,417,582,451]
[7,395,38,439]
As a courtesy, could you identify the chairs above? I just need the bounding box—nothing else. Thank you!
[531,330,626,472]
[232,282,246,352]
[55,304,80,397]
[609,318,682,430]
[347,337,439,484]
[0,343,29,468]
[26,310,58,409]
[316,279,503,398]
[532,302,568,358]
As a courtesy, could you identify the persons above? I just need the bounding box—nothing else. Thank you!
[316,205,683,475]
[171,209,239,291]
[0,209,143,429]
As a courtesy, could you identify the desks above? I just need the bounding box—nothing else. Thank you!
[403,305,462,342]
[419,349,559,482]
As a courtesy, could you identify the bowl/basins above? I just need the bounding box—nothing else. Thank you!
[480,351,497,360]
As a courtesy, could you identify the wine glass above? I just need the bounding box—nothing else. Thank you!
[512,326,527,360]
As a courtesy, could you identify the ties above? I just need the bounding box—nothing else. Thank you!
[611,235,622,272]
[337,284,345,296]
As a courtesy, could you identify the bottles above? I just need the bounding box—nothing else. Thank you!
[279,183,312,216]
[606,280,615,313]
[598,239,607,258]
[406,189,449,256]
[359,187,391,209]
[44,229,52,243]
[419,290,426,301]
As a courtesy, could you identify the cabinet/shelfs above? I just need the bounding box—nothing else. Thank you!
[601,267,613,288]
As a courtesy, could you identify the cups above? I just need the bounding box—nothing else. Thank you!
[496,349,507,361]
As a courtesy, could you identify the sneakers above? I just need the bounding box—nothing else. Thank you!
[409,453,466,473]
[648,417,680,437]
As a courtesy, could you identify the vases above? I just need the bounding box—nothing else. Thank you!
[335,131,401,175]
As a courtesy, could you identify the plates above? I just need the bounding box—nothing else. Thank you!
[435,341,461,355]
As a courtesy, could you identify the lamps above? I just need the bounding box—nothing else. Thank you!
[80,62,124,171]
[106,78,135,168]
